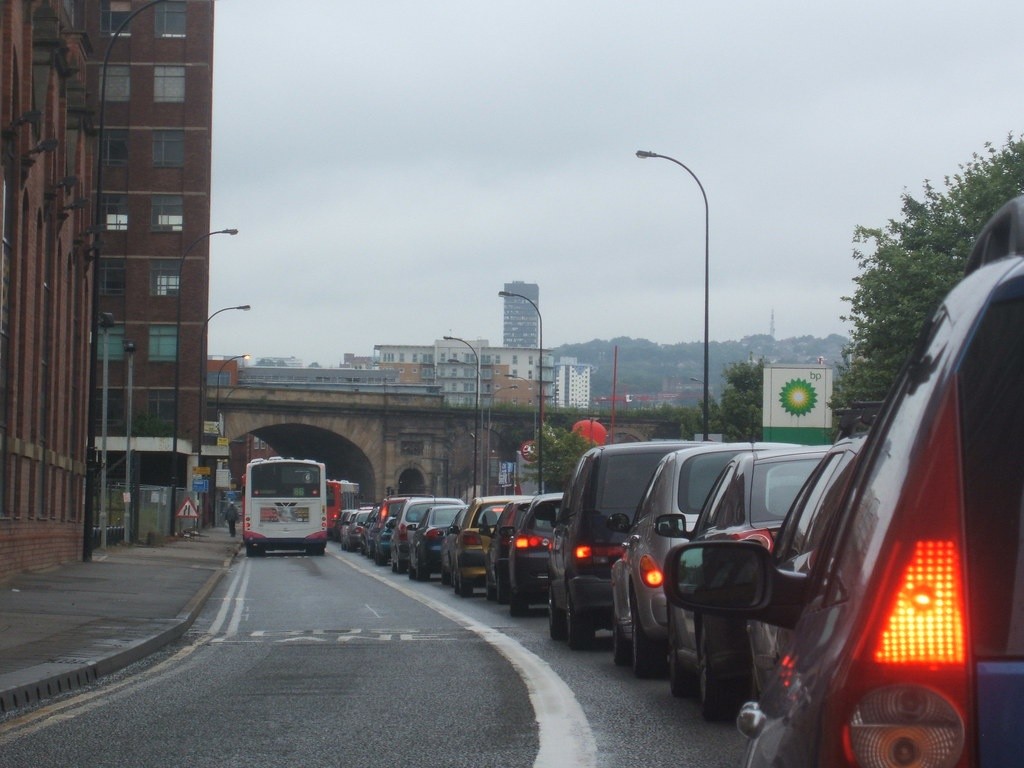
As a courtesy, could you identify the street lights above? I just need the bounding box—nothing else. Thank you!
[485,386,519,498]
[121,337,138,547]
[212,355,251,528]
[446,358,483,497]
[223,385,252,437]
[495,289,544,495]
[441,335,480,501]
[504,373,537,442]
[99,312,115,552]
[170,227,240,540]
[194,305,252,530]
[635,150,713,443]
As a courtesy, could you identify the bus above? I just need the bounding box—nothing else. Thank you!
[327,478,359,541]
[244,457,327,557]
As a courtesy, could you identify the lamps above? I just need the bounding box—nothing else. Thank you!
[22,136,58,161]
[88,240,105,252]
[52,174,78,193]
[12,108,42,131]
[62,198,89,210]
[85,223,108,237]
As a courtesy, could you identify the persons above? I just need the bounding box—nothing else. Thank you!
[225,502,239,537]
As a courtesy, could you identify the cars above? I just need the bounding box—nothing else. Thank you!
[448,494,529,599]
[477,497,534,606]
[498,490,564,616]
[736,430,872,701]
[605,438,806,682]
[651,444,834,723]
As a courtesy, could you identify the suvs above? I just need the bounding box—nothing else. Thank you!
[531,437,700,653]
[663,194,1024,768]
[331,493,468,588]
